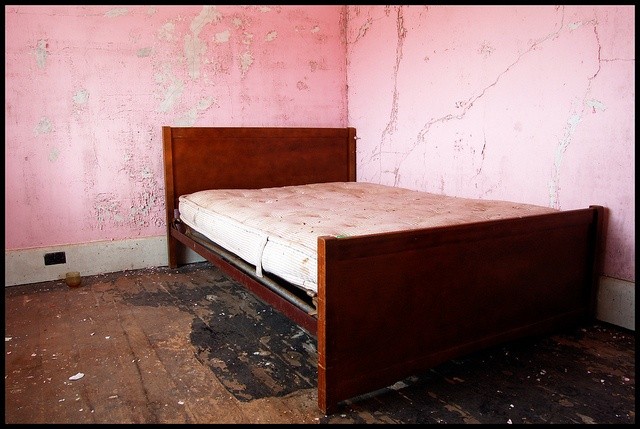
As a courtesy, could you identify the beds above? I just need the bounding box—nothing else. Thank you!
[162,126,604,409]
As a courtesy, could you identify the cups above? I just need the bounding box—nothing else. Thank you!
[65,272,82,288]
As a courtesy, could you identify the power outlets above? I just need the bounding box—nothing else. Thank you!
[45,252,66,265]
